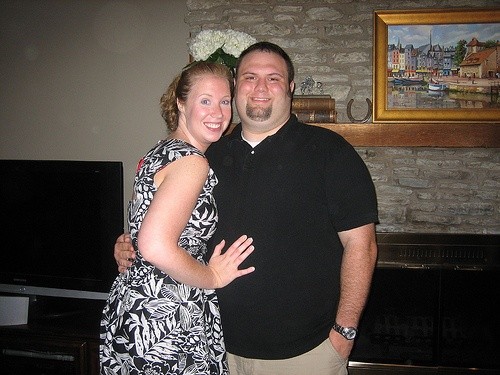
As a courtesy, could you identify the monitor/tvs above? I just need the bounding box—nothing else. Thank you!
[0,159,125,301]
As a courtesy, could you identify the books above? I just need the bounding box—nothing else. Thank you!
[290,110,337,124]
[292,97,335,110]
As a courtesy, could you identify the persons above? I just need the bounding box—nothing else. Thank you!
[114,40,380,375]
[100,62,254,374]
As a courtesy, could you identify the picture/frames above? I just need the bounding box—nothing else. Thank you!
[370,7,500,124]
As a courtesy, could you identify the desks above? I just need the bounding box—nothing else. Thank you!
[0,292,107,375]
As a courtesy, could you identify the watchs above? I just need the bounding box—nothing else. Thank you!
[332,322,358,340]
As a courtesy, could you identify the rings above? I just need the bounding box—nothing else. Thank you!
[117,259,123,266]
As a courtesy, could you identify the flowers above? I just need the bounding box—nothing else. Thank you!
[186,27,260,79]
